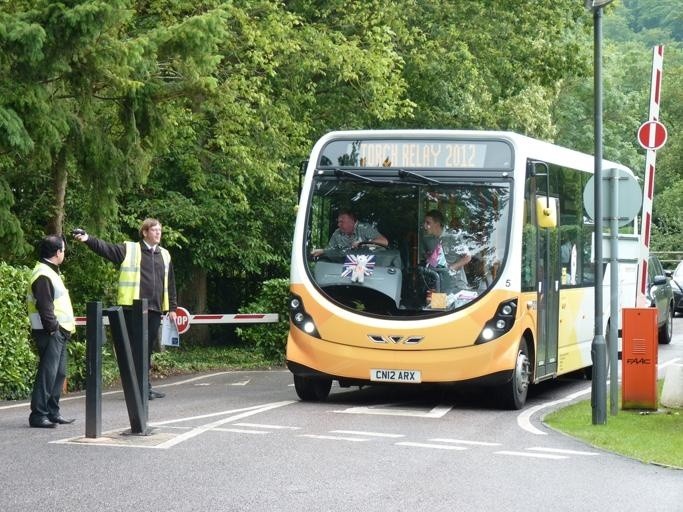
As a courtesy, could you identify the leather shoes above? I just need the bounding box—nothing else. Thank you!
[48,412,75,424]
[29,415,57,428]
[150,393,155,400]
[150,388,165,398]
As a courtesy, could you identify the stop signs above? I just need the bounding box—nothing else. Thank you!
[165,306,191,335]
[636,120,668,151]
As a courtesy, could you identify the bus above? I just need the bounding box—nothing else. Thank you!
[283,127,640,411]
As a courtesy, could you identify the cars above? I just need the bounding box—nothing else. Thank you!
[664,260,683,313]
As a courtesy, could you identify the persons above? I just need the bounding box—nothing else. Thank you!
[311,209,388,257]
[26,235,77,428]
[423,210,471,295]
[72,217,177,400]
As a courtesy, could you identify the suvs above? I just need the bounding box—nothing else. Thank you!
[647,253,676,345]
